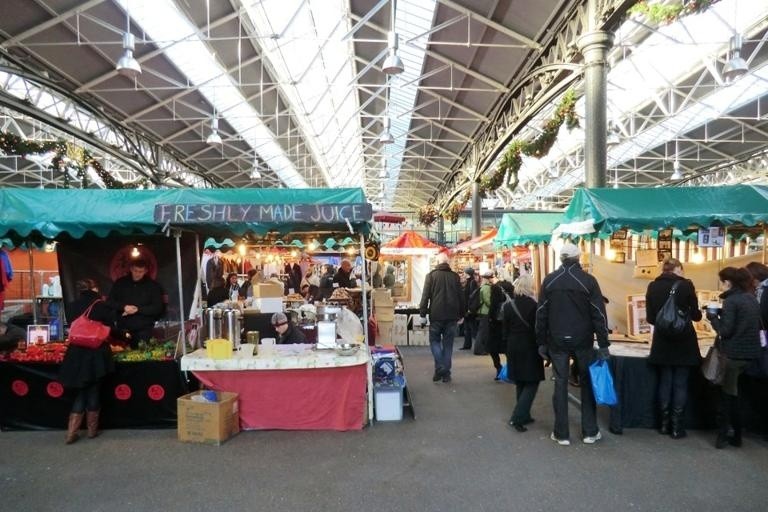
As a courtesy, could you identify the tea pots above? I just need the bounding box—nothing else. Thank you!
[202,307,243,350]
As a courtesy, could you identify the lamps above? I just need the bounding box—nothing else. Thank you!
[378,147,390,179]
[380,0,405,73]
[667,140,687,181]
[111,0,145,80]
[204,117,224,146]
[129,238,143,259]
[249,157,261,180]
[378,86,394,144]
[721,34,750,75]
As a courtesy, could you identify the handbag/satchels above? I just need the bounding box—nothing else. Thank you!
[69,316,113,351]
[701,347,726,385]
[654,290,692,345]
[759,329,767,347]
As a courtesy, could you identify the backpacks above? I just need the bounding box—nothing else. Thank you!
[468,286,485,313]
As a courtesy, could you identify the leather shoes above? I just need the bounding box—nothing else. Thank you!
[507,420,527,432]
[523,418,533,425]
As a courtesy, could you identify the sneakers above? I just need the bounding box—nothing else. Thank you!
[443,377,451,382]
[551,432,570,446]
[433,367,447,381]
[581,431,602,444]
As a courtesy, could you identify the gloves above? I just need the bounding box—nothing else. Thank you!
[597,347,612,360]
[538,346,550,360]
[706,313,716,320]
[419,317,428,329]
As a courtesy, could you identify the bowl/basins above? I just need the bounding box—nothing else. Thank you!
[333,342,361,356]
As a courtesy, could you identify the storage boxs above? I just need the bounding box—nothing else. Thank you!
[252,281,282,297]
[370,288,394,345]
[407,314,430,331]
[176,389,240,446]
[253,298,282,314]
[372,383,404,423]
[391,314,407,346]
[408,329,430,346]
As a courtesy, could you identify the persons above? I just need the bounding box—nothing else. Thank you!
[270,312,308,345]
[419,252,467,383]
[205,248,395,308]
[0,322,28,352]
[458,265,516,381]
[705,266,760,449]
[109,258,169,349]
[535,243,612,446]
[747,260,768,354]
[61,278,115,443]
[502,271,546,433]
[644,257,703,439]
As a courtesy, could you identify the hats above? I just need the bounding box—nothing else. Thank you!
[483,271,494,277]
[559,243,582,259]
[270,312,289,326]
[464,268,474,274]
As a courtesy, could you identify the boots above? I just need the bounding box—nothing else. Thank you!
[65,413,83,445]
[657,388,741,448]
[86,410,103,439]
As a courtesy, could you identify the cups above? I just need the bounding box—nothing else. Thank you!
[246,330,259,356]
[238,342,256,359]
[260,335,276,354]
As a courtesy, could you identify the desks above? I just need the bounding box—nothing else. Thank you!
[180,341,369,430]
[590,332,768,439]
[0,342,181,431]
